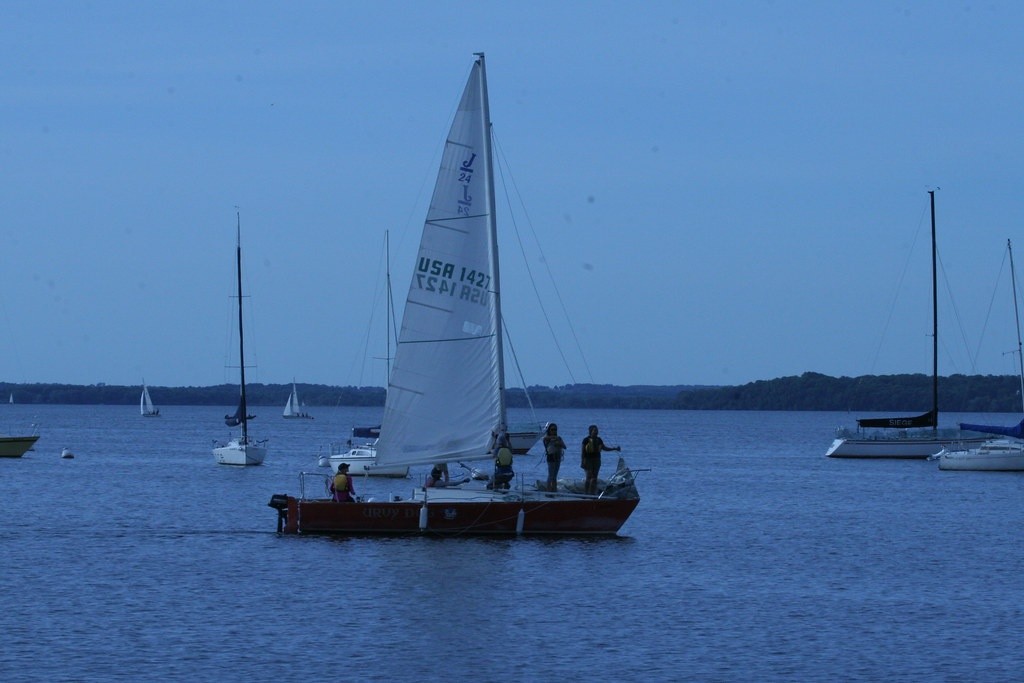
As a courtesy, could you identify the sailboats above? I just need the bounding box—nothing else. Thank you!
[281,377,315,419]
[138,374,162,419]
[269,47,645,539]
[206,202,273,468]
[928,238,1024,471]
[823,188,1000,457]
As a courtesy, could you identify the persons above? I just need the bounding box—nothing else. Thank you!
[431,463,448,483]
[330,463,355,502]
[581,425,619,494]
[487,434,514,489]
[543,423,566,489]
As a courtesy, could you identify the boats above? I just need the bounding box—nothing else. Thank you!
[324,443,410,479]
[61,447,75,461]
[0,431,39,458]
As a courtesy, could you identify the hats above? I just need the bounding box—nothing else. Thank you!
[589,425,595,433]
[337,463,350,470]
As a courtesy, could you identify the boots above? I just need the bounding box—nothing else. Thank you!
[553,481,557,492]
[546,481,554,492]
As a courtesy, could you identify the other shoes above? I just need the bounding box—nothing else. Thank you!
[586,490,597,494]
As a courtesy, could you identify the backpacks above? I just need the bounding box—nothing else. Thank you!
[496,447,512,466]
[546,439,559,454]
[333,474,348,492]
